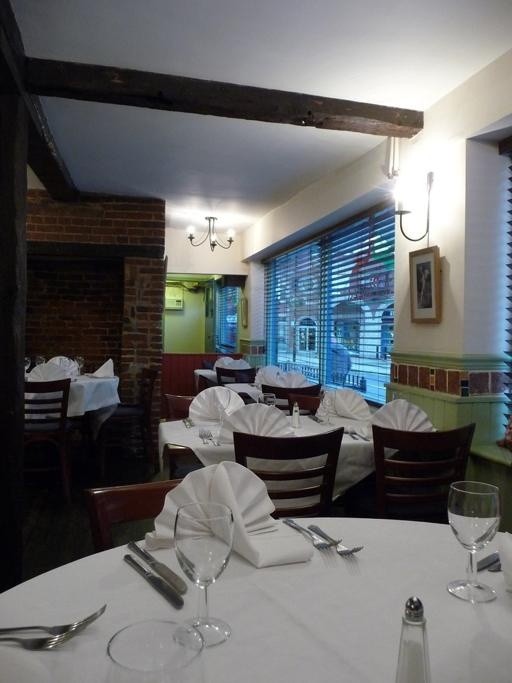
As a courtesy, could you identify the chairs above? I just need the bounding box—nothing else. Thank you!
[215,366,257,388]
[98,365,159,457]
[24,378,75,498]
[369,421,477,524]
[260,382,321,416]
[82,478,182,553]
[231,427,344,523]
[286,389,319,417]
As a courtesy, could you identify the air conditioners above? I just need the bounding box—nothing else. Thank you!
[165,298,185,311]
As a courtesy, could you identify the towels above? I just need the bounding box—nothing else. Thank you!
[92,358,115,380]
[278,369,306,386]
[366,398,437,440]
[316,388,373,420]
[215,402,298,448]
[28,364,75,384]
[212,357,235,371]
[188,385,244,422]
[253,365,282,385]
[143,458,315,572]
[48,356,80,379]
[226,359,251,370]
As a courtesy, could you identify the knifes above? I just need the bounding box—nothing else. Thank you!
[127,542,187,593]
[124,554,184,610]
[476,552,502,577]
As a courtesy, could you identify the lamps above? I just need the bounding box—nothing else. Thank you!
[186,214,235,253]
[387,167,435,245]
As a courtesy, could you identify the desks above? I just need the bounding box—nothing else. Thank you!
[23,372,117,456]
[156,413,438,492]
[0,516,512,682]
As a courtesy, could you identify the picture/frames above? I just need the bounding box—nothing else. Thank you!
[407,247,443,326]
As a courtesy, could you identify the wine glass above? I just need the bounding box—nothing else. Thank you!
[319,386,336,427]
[23,355,84,381]
[172,502,233,647]
[258,393,276,405]
[108,621,202,683]
[447,479,499,602]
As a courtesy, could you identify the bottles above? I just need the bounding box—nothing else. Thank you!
[292,401,300,428]
[395,595,434,683]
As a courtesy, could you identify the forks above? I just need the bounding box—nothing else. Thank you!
[281,518,343,551]
[0,604,109,634]
[0,629,88,651]
[308,525,364,556]
[199,430,221,446]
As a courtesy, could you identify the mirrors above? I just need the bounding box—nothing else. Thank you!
[163,270,249,357]
